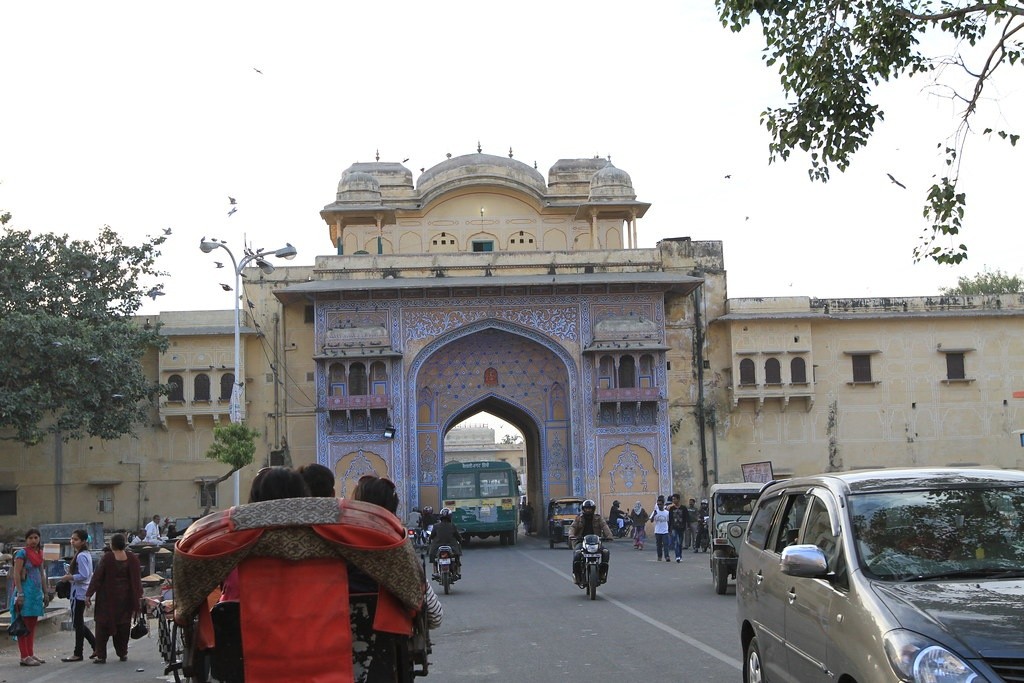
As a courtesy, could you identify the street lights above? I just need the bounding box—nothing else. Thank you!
[199,239,297,506]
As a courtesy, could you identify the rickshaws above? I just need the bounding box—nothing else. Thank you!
[169,499,436,683]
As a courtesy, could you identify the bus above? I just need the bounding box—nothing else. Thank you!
[441,461,521,545]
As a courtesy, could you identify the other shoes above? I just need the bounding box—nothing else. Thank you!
[120,656,127,660]
[61,655,83,661]
[30,655,46,663]
[93,659,105,663]
[20,656,40,666]
[89,652,98,658]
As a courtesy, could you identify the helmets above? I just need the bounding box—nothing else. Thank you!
[581,500,596,514]
[423,505,433,514]
[439,507,452,520]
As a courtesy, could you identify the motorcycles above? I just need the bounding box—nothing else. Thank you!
[604,508,636,540]
[406,527,434,554]
[431,541,464,595]
[697,520,711,552]
[571,536,612,600]
[548,496,584,549]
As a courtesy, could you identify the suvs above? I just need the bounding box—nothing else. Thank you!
[708,482,766,595]
[733,466,1024,683]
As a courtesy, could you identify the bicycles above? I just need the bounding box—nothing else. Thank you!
[163,611,192,683]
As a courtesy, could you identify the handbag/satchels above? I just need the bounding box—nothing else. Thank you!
[6,601,30,635]
[130,609,148,639]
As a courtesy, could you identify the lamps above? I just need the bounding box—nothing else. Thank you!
[384,425,396,439]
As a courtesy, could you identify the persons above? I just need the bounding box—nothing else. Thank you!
[246,465,312,503]
[85,533,144,664]
[292,463,335,498]
[631,501,648,551]
[419,505,437,531]
[102,514,178,562]
[9,528,50,666]
[517,498,573,541]
[649,499,672,562]
[668,494,691,562]
[569,499,613,583]
[428,508,464,581]
[610,499,628,537]
[407,507,424,543]
[348,475,444,631]
[60,528,98,662]
[651,496,760,553]
